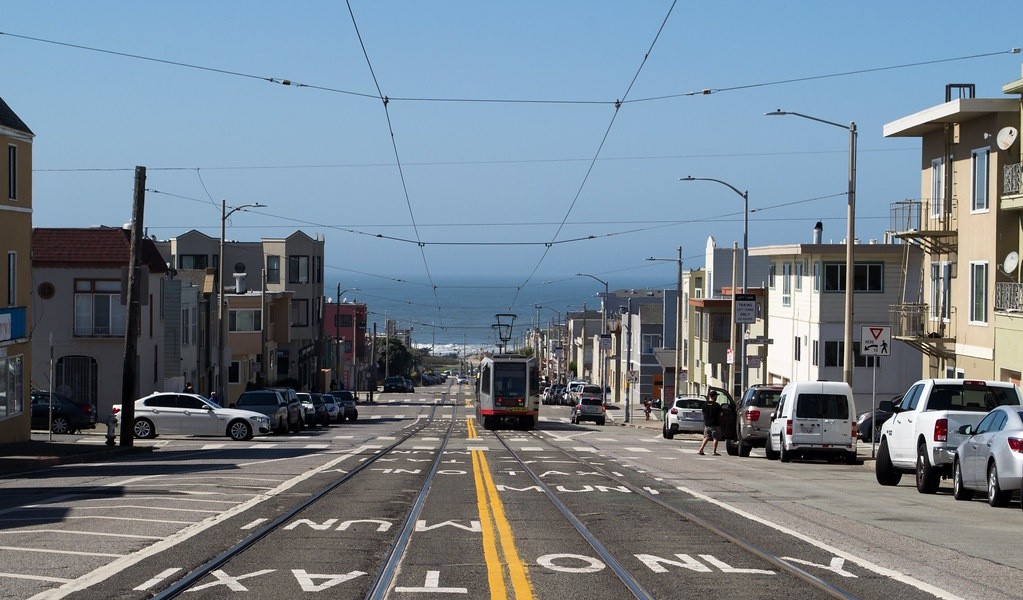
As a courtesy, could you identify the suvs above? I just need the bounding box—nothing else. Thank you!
[663,394,704,439]
[570,397,606,425]
[704,385,785,455]
[571,384,603,405]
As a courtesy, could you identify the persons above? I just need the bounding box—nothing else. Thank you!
[643,398,651,420]
[245,378,255,391]
[339,381,344,390]
[329,379,336,391]
[183,383,195,394]
[698,391,723,456]
[208,392,218,404]
[187,386,192,393]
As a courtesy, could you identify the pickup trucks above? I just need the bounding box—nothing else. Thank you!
[875,379,1023,494]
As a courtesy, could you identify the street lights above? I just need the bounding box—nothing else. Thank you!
[542,304,587,385]
[218,204,268,403]
[590,294,631,423]
[679,176,749,397]
[642,257,681,395]
[337,288,362,384]
[767,111,856,388]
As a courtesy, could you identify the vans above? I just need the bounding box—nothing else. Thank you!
[766,380,856,464]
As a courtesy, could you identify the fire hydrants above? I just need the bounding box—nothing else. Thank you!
[366,393,370,401]
[106,414,119,446]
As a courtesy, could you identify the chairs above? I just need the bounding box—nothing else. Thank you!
[937,389,952,406]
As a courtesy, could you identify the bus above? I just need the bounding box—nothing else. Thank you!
[475,353,540,429]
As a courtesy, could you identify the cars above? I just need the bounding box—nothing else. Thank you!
[423,371,468,385]
[953,405,1023,505]
[856,393,903,442]
[234,387,359,434]
[116,393,272,440]
[541,382,588,404]
[385,376,415,393]
[30,392,96,433]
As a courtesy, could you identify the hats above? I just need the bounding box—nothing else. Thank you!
[710,390,720,396]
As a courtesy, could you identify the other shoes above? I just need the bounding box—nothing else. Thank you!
[713,453,721,456]
[697,452,705,455]
